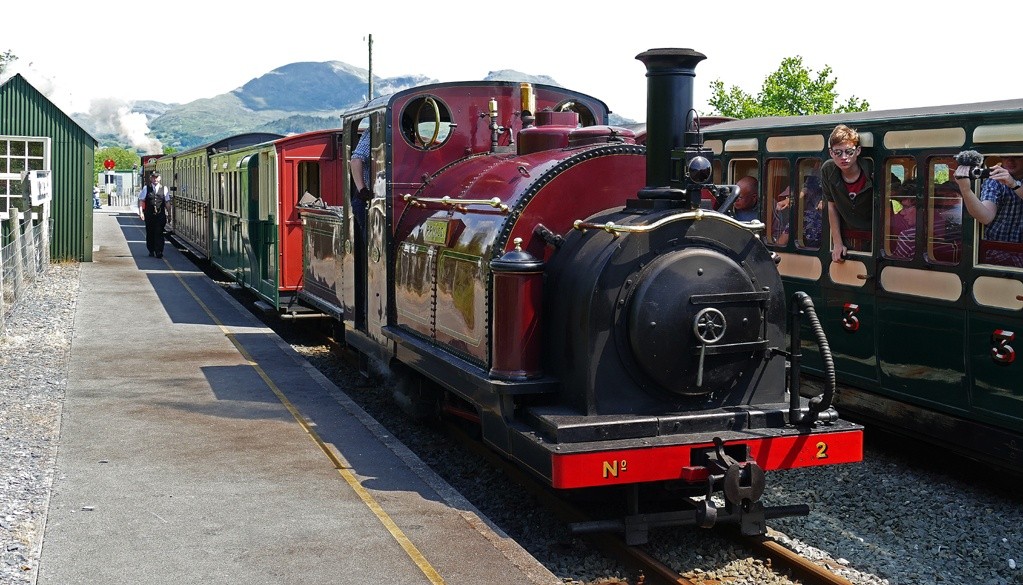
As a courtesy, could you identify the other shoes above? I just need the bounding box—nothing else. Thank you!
[149,252,154,256]
[156,251,163,258]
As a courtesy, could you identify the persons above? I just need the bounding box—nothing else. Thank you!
[92,183,103,209]
[349,127,373,233]
[138,172,171,259]
[822,125,884,264]
[732,164,1022,271]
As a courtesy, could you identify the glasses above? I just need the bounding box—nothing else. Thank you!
[831,148,857,157]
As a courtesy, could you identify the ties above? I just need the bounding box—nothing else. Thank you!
[153,184,157,193]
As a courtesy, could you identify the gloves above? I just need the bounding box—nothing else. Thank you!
[358,187,370,199]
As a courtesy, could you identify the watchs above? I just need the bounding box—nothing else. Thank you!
[1012,180,1021,191]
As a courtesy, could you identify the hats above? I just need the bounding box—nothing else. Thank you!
[149,170,160,176]
[778,185,790,196]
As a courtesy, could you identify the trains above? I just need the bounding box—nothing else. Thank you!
[138,33,867,548]
[615,96,1023,481]
